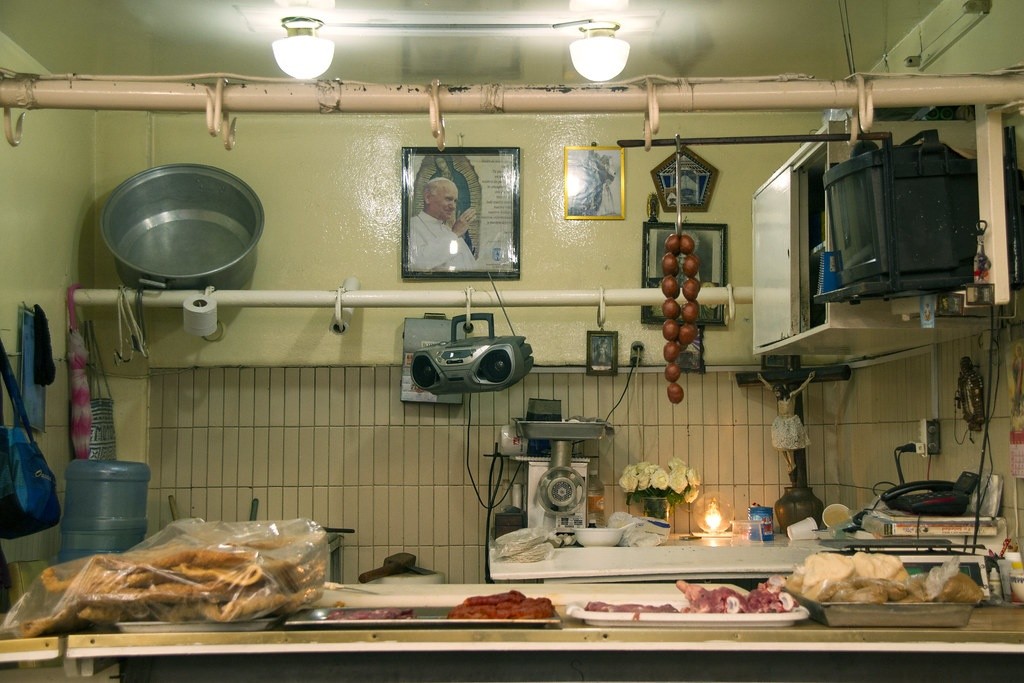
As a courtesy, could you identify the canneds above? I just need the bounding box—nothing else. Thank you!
[747,506,774,542]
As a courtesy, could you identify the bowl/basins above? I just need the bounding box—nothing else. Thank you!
[1009,574,1024,605]
[571,524,626,547]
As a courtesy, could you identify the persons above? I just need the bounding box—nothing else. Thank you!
[757,371,815,473]
[408,178,477,271]
[594,347,610,366]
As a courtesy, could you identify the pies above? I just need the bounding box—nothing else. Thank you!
[5,529,330,639]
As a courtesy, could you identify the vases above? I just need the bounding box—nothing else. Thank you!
[641,496,669,522]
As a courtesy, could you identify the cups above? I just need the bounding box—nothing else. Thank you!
[822,503,853,528]
[787,518,818,542]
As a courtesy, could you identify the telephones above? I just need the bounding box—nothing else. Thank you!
[881,471,979,516]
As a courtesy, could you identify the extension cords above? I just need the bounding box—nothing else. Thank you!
[910,441,924,454]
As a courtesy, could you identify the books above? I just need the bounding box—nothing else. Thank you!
[812,508,1008,556]
[970,472,1004,517]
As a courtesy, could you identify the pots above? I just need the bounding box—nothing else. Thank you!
[101,162,264,292]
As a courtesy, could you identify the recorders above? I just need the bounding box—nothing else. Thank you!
[410,270,534,395]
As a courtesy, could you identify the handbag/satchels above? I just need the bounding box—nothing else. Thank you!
[0,422,61,540]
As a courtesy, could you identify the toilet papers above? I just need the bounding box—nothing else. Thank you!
[182,293,218,337]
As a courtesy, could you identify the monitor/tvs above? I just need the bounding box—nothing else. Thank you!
[822,142,979,287]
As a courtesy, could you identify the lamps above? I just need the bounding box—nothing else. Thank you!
[568,21,631,82]
[272,15,335,80]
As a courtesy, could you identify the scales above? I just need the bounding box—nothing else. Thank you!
[819,538,991,602]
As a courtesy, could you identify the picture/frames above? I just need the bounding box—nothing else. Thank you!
[650,145,719,213]
[641,221,728,327]
[587,330,619,376]
[400,145,522,279]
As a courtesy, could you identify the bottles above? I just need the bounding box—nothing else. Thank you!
[587,519,597,528]
[53,461,149,563]
[989,553,1021,604]
[501,479,511,506]
[587,470,607,528]
[974,220,989,284]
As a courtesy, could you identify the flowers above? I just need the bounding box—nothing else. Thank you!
[619,460,702,518]
